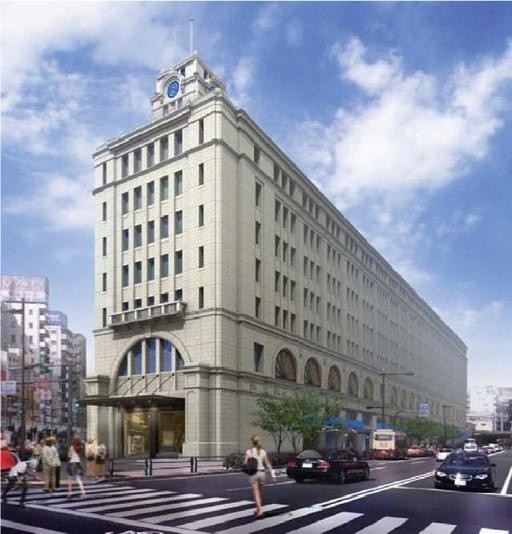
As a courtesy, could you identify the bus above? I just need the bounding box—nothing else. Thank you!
[371,428,409,459]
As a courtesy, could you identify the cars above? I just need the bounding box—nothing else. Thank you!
[434,450,496,491]
[285,448,369,483]
[407,438,503,461]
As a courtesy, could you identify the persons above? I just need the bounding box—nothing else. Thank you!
[1,426,110,508]
[243,435,278,517]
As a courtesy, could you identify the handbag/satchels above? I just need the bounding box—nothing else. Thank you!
[241,449,258,475]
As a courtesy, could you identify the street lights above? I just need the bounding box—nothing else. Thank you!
[443,403,451,436]
[24,362,74,448]
[366,369,415,427]
